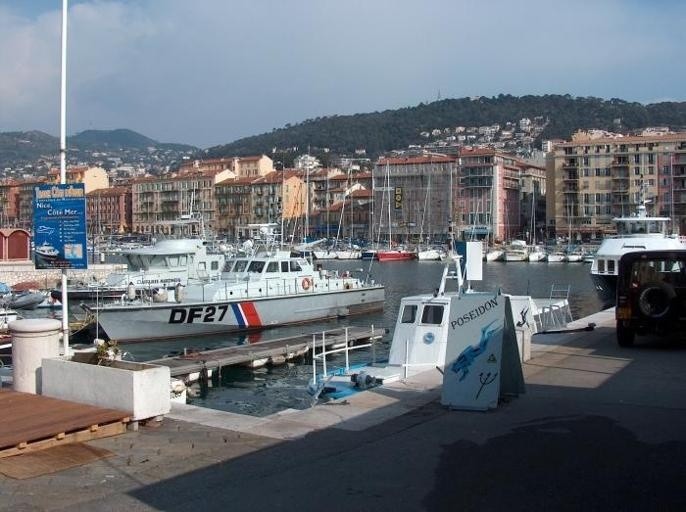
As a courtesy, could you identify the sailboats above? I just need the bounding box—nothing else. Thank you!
[290,160,440,262]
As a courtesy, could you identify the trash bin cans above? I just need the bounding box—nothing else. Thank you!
[8,318,62,395]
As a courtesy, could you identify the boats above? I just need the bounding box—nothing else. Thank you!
[483,184,594,263]
[306,240,573,403]
[51,215,225,300]
[36,240,59,260]
[590,174,686,298]
[0,291,45,353]
[90,223,385,344]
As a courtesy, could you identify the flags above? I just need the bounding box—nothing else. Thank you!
[455,240,483,281]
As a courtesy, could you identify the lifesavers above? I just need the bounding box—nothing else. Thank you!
[302,278,311,290]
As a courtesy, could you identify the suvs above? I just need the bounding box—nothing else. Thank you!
[616,250,686,347]
